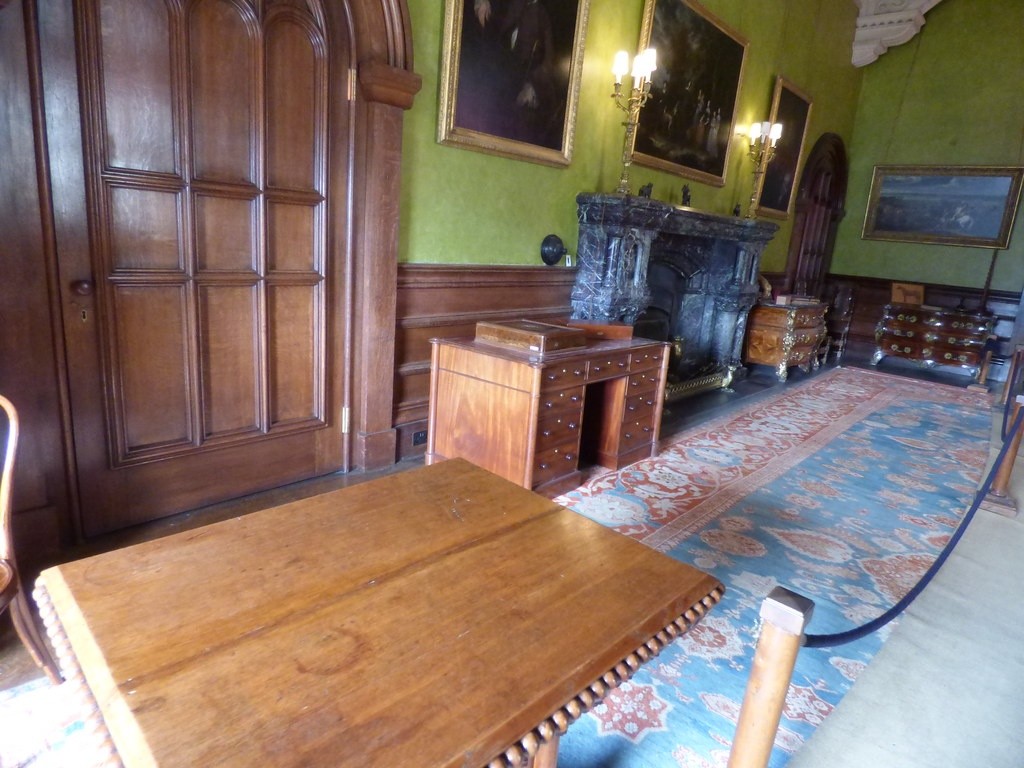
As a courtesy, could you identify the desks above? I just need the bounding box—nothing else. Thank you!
[30,455,727,768]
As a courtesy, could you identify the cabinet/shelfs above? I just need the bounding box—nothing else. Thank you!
[742,301,827,382]
[424,317,673,499]
[870,301,995,378]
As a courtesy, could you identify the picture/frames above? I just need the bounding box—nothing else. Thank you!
[623,0,750,188]
[748,74,814,221]
[436,0,590,171]
[860,165,1024,249]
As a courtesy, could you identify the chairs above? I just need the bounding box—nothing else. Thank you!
[0,395,64,686]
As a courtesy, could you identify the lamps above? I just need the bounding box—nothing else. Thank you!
[745,122,783,219]
[611,48,658,199]
